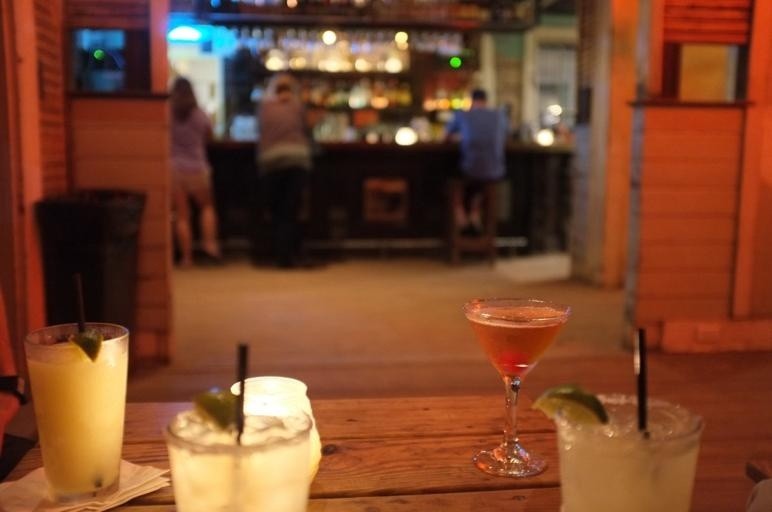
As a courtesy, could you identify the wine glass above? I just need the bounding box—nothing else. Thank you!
[463,299,572,477]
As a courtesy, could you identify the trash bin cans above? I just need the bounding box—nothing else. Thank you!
[34,190,144,370]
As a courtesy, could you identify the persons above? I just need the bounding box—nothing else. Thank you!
[253,71,313,181]
[169,75,226,268]
[440,88,514,234]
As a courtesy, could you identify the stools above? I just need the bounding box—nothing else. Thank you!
[447,180,499,272]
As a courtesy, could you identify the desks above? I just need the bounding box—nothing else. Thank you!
[1,389,563,510]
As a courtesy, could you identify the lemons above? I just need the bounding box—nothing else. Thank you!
[69,327,104,363]
[192,386,239,433]
[533,382,610,430]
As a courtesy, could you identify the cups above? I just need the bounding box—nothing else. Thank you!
[23,322,129,499]
[556,395,703,512]
[164,405,313,512]
